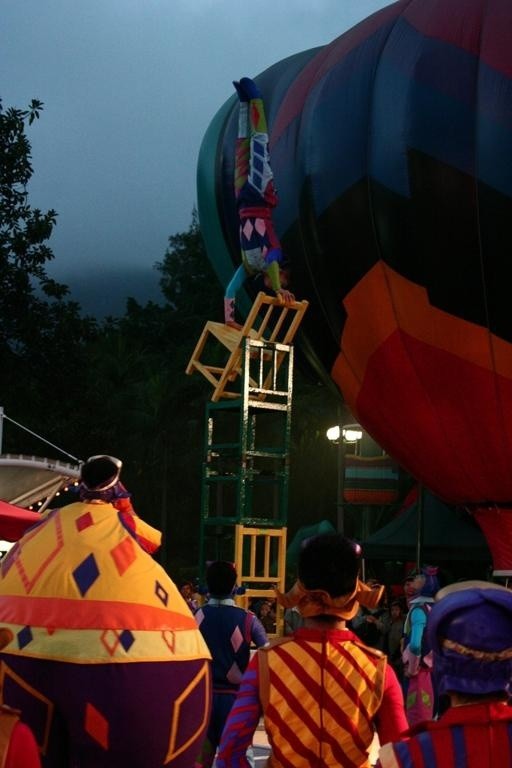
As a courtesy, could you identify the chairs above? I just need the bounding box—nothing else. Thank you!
[234,523,289,641]
[201,337,296,475]
[186,294,309,402]
[194,475,289,596]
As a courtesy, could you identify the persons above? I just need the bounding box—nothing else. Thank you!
[370,576,512,767]
[398,563,443,729]
[188,558,272,768]
[178,581,407,670]
[178,535,410,767]
[221,75,299,334]
